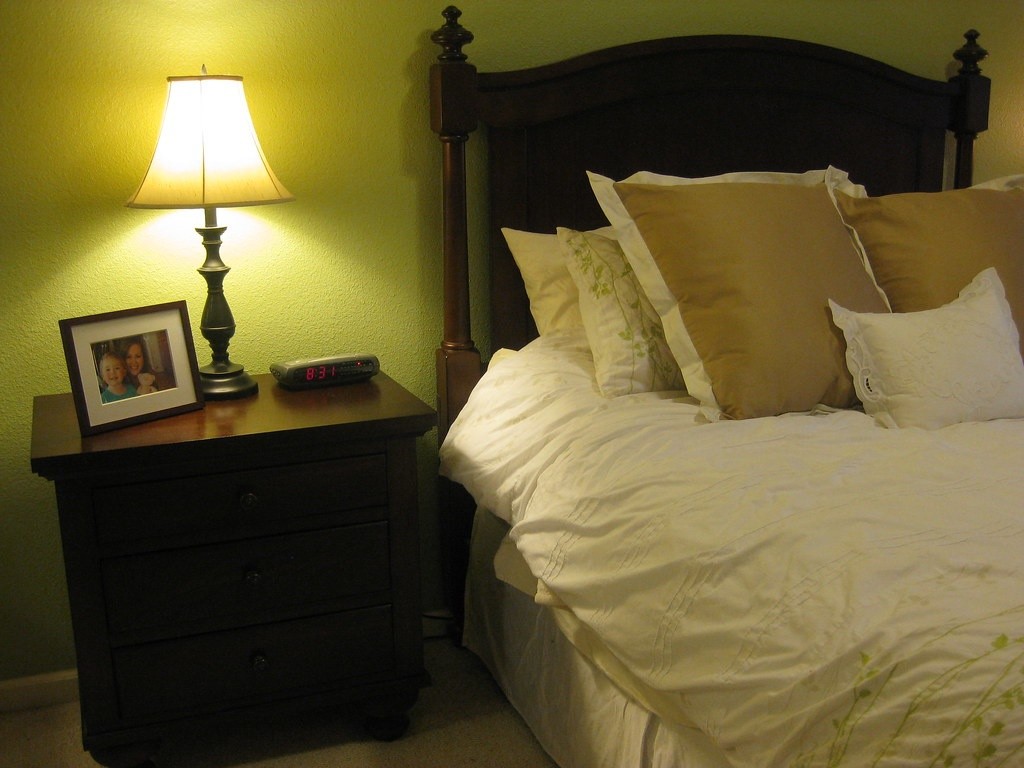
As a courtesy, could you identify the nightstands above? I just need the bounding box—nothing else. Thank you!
[29,369,440,768]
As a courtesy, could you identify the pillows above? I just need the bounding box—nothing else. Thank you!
[500,225,619,337]
[556,227,686,397]
[824,165,1024,359]
[585,170,893,422]
[828,267,1024,428]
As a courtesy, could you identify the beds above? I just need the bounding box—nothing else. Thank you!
[430,4,1024,768]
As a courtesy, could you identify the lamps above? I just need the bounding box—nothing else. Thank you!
[126,63,297,401]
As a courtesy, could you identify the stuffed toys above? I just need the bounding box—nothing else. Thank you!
[137,372,156,394]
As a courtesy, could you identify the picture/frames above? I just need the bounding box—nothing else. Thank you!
[59,300,206,437]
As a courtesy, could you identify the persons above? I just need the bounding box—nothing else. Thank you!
[100,342,160,403]
